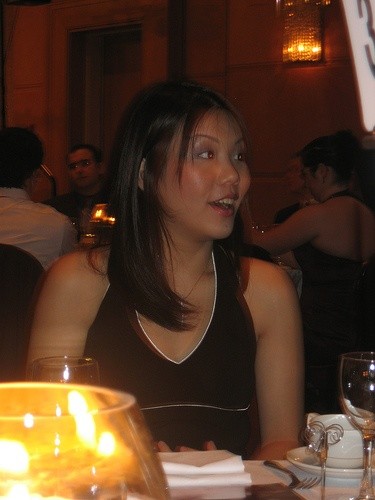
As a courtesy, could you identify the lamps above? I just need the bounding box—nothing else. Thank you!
[275,0,332,65]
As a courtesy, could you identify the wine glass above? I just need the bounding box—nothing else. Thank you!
[339,352,375,500]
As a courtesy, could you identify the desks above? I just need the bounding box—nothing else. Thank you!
[171,460,360,500]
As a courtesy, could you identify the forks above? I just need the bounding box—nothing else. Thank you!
[264,460,322,490]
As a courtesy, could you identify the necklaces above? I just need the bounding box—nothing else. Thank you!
[181,266,209,322]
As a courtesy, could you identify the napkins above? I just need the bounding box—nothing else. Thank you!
[158,449,255,487]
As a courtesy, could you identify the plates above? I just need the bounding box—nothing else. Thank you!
[286,445,375,480]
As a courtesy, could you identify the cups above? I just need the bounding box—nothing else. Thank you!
[310,414,365,468]
[26,355,101,388]
[0,381,171,499]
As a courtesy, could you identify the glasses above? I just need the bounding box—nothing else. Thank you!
[68,159,100,170]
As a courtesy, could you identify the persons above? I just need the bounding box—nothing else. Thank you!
[27,80,305,457]
[0,127,77,270]
[246,130,375,413]
[45,143,106,239]
